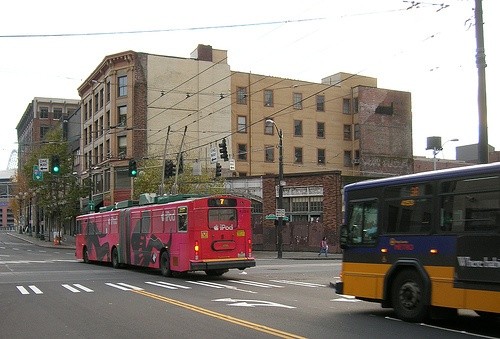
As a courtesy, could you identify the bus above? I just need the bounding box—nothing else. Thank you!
[334,162,500,323]
[75,193,256,277]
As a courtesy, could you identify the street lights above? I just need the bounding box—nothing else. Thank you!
[433,138,459,171]
[265,119,283,257]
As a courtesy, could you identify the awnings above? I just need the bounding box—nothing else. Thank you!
[83,200,103,212]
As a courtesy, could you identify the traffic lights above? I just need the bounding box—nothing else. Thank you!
[166,159,176,177]
[52,156,60,174]
[216,163,222,177]
[219,139,229,161]
[129,159,137,178]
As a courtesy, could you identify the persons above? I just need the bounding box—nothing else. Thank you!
[318,237,328,258]
